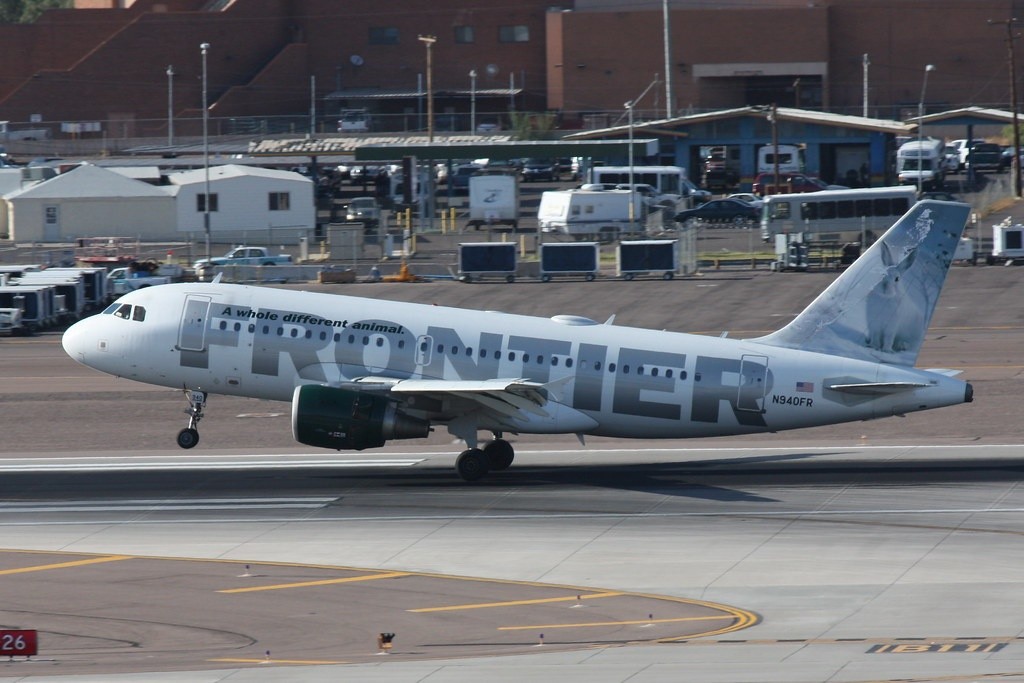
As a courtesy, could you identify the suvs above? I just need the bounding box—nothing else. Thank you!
[338,108,374,133]
[752,168,824,197]
[946,137,1024,174]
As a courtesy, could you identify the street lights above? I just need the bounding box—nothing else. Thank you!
[198,40,212,255]
[468,69,478,136]
[165,65,178,149]
[916,65,937,199]
[624,101,635,222]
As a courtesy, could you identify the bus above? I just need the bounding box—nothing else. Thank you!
[760,183,919,246]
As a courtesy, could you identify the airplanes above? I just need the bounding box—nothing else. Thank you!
[62,198,974,482]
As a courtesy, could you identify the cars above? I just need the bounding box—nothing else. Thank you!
[787,176,849,190]
[195,245,293,267]
[674,196,764,226]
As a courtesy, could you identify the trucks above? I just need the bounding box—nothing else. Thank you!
[537,183,681,242]
[589,164,713,200]
[897,140,947,191]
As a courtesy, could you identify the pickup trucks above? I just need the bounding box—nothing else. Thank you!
[105,267,171,300]
